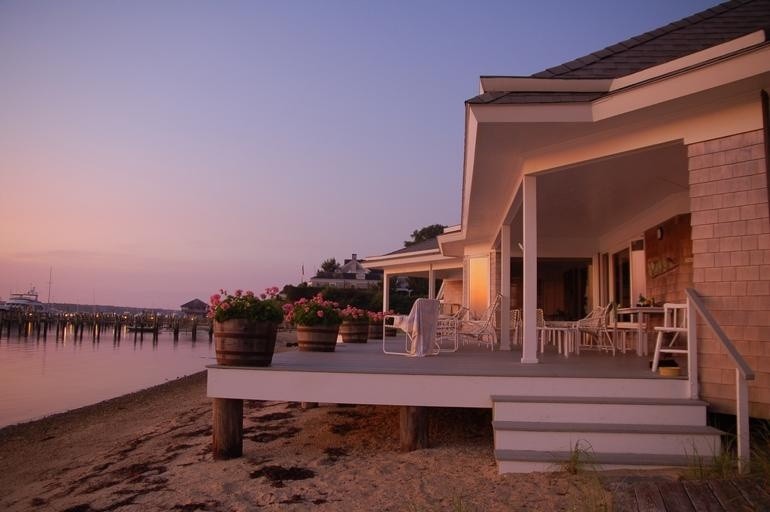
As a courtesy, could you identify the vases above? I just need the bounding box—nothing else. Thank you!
[209,320,279,369]
[291,320,342,355]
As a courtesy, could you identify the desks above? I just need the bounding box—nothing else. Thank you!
[617,307,670,357]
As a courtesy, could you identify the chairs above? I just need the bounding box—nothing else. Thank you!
[651,302,689,373]
[382,296,617,360]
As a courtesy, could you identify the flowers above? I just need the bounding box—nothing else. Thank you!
[205,284,284,329]
[284,292,344,324]
[336,302,400,345]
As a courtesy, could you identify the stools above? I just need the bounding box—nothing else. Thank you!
[608,321,647,354]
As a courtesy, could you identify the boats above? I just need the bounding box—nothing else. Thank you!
[0,283,59,313]
[128,323,154,332]
